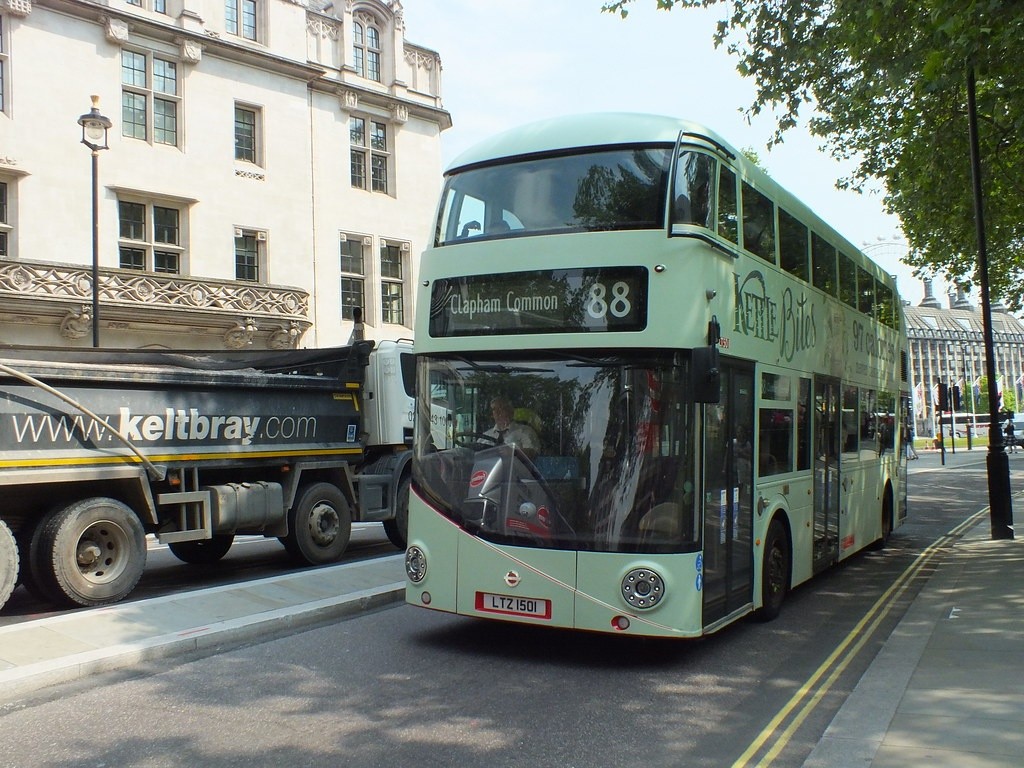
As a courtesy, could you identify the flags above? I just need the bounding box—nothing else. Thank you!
[954,379,964,408]
[915,382,924,415]
[973,376,981,407]
[996,377,1004,410]
[1016,375,1024,400]
[931,383,940,406]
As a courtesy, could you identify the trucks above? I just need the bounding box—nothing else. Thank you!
[1,335,459,617]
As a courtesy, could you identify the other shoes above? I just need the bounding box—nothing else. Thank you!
[1015,452,1017,453]
[1009,452,1012,454]
[906,455,918,460]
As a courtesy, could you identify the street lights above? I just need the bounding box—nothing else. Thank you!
[76,91,112,348]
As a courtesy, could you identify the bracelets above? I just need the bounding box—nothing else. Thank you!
[722,470,725,473]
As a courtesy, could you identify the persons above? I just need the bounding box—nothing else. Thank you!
[720,424,752,482]
[1004,419,1018,454]
[907,425,919,460]
[461,220,509,237]
[475,394,542,459]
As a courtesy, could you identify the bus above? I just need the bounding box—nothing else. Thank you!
[932,413,1009,439]
[404,108,913,641]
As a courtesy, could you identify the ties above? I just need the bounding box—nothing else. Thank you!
[496,428,509,446]
